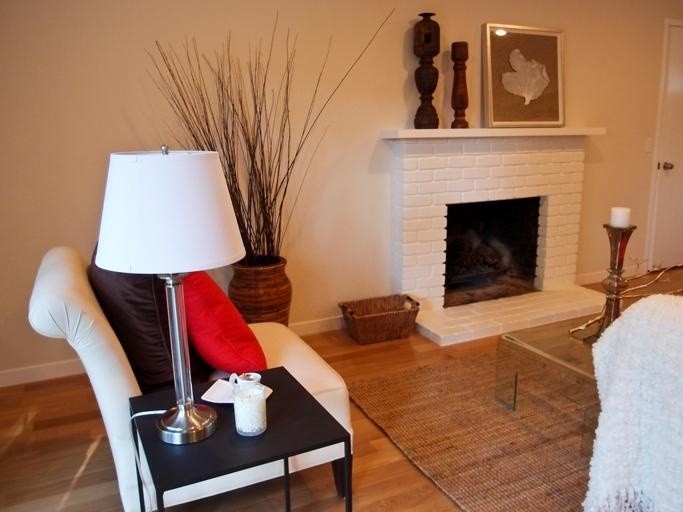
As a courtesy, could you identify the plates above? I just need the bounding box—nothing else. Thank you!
[200,378,274,404]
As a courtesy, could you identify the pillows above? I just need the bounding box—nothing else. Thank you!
[85,246,268,393]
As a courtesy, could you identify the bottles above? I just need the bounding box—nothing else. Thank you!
[229,372,268,437]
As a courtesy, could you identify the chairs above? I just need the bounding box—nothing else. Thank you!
[25,245,352,511]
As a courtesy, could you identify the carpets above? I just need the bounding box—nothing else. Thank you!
[346,325,601,512]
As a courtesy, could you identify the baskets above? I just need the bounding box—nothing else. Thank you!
[337,293,421,345]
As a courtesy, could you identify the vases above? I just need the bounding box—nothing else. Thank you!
[228,255,293,328]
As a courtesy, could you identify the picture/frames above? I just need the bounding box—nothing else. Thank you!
[480,21,567,128]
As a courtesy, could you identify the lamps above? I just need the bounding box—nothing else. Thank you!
[93,145,250,446]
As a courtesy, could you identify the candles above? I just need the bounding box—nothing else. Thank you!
[609,206,632,228]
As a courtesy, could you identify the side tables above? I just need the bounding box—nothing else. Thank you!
[127,365,351,511]
